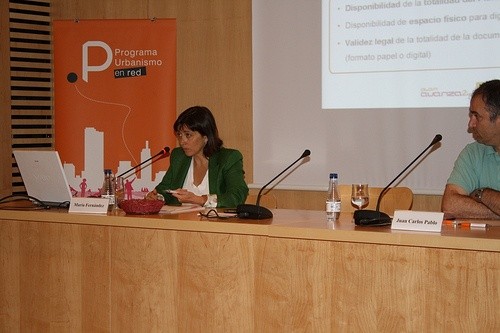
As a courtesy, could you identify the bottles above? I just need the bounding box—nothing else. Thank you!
[324,173,341,222]
[102,169,115,212]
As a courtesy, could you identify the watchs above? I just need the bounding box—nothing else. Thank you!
[475,188,484,201]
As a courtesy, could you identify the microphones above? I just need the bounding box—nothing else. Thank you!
[111,146,170,182]
[353,134,443,226]
[236,149,311,220]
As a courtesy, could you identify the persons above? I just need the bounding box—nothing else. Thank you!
[441,79,500,220]
[145,106,248,208]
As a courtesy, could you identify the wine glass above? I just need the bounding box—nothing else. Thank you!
[351,183,369,209]
[112,177,124,210]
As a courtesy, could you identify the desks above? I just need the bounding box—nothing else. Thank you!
[1,205,500,333]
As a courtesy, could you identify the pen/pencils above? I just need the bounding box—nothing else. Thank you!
[165,188,179,195]
[440,220,457,224]
[457,221,488,228]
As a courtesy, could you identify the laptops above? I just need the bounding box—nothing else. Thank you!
[13,150,73,207]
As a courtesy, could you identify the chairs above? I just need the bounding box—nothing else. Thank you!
[340,186,413,214]
